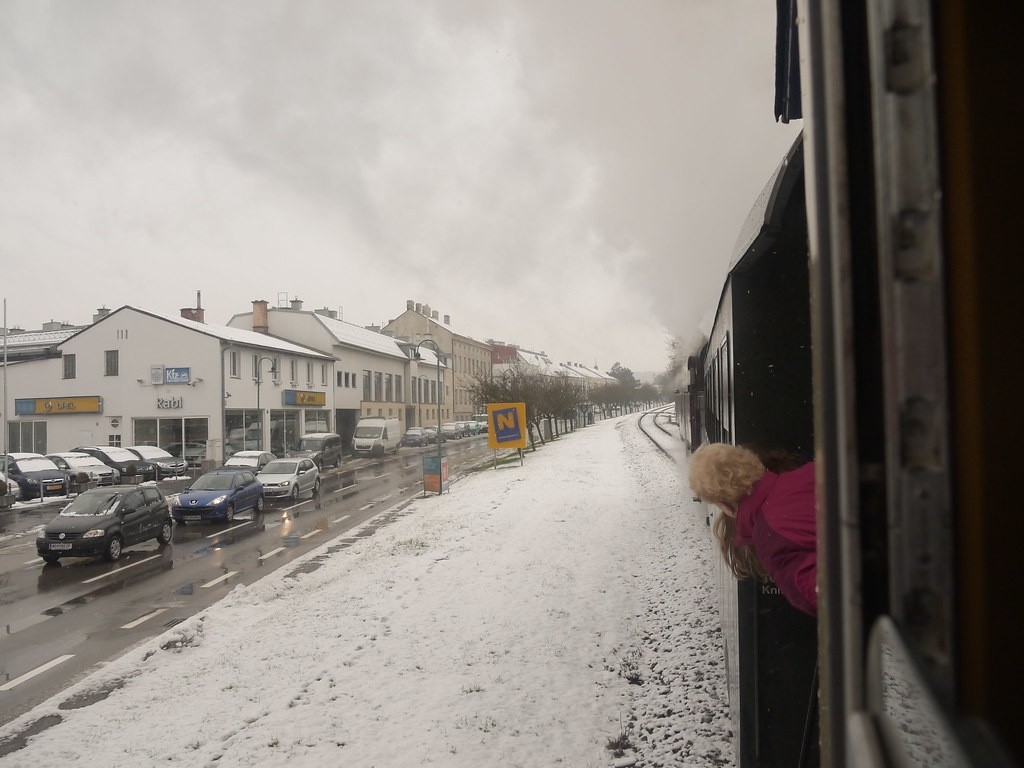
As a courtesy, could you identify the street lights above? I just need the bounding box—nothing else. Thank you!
[409,339,441,456]
[257,356,278,450]
[503,369,515,401]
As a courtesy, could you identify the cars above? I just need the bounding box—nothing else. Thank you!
[4,475,20,501]
[225,451,278,469]
[427,421,480,444]
[404,426,428,446]
[170,470,265,522]
[179,440,235,466]
[126,447,188,479]
[45,452,120,489]
[256,458,321,500]
[36,484,172,562]
[6,453,68,500]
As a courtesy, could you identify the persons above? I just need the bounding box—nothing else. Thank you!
[685,440,817,623]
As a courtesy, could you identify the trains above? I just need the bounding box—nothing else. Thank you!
[674,2,1024,768]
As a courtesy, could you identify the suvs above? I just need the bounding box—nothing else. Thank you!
[70,445,154,481]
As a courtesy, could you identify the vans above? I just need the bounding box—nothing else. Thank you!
[288,433,342,472]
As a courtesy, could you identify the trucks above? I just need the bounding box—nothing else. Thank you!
[351,416,401,458]
[473,415,489,433]
